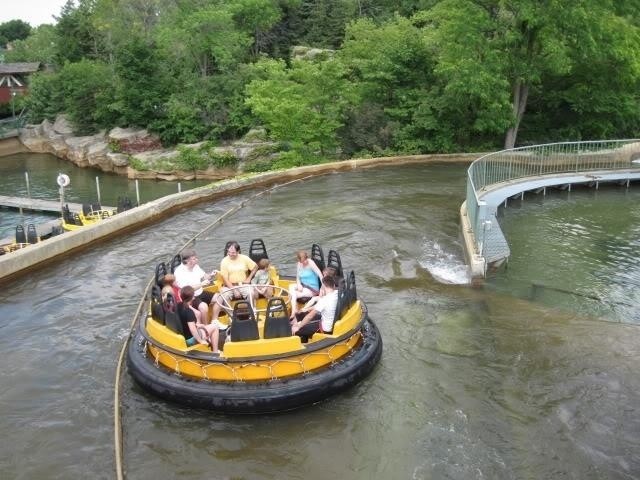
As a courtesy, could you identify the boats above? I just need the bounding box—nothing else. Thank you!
[0,196,136,258]
[126,238,382,417]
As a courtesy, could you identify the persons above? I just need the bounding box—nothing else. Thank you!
[292,276,345,343]
[288,250,325,320]
[160,273,202,324]
[219,241,259,320]
[176,286,220,352]
[172,249,229,331]
[250,258,273,310]
[289,266,338,333]
